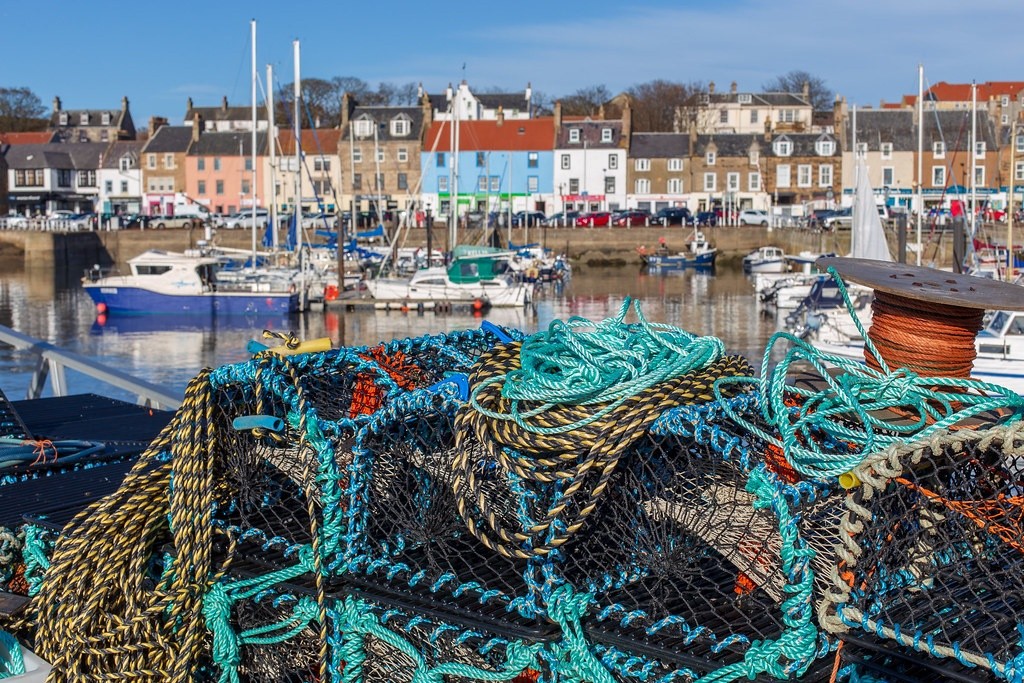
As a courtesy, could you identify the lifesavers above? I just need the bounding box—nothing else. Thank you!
[323,285,340,301]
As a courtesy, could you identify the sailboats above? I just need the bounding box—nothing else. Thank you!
[80,17,574,313]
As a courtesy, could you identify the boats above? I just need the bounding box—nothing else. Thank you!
[635,216,718,271]
[760,63,1024,394]
[742,245,792,276]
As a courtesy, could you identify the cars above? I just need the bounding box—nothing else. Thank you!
[103,193,1024,233]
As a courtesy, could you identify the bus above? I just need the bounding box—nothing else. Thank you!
[6,185,102,217]
